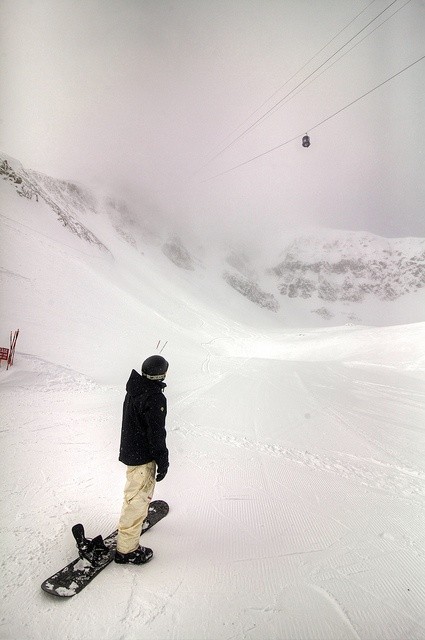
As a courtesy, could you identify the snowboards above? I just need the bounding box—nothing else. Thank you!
[40,500,170,598]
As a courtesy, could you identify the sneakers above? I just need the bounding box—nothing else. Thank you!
[143,520,149,529]
[115,547,153,565]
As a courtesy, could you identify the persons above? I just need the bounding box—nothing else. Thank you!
[115,356,169,564]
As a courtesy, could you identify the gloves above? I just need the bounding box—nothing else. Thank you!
[155,449,169,481]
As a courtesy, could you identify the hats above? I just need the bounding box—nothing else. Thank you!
[142,356,168,380]
